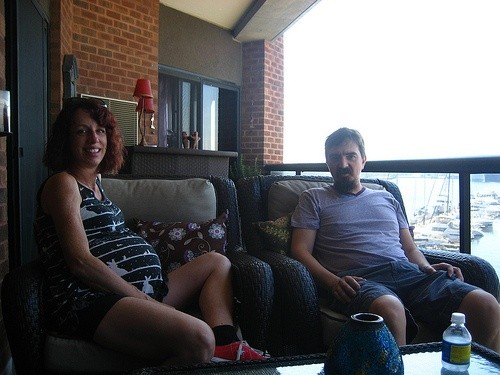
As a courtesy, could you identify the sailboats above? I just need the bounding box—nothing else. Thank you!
[410,174,499,251]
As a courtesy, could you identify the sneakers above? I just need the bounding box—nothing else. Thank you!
[211,341,271,363]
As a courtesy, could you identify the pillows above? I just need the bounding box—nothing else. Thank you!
[252,213,293,256]
[128,209,229,266]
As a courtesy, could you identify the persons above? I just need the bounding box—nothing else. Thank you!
[291,129,500,356]
[35,99,272,366]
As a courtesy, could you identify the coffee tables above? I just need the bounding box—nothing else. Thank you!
[136,341,500,375]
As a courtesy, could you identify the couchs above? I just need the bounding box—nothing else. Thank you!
[238,175,500,357]
[0,176,275,375]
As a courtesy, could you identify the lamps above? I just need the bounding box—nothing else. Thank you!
[133,79,160,148]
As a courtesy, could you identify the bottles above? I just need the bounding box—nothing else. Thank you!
[440,312,472,375]
[323,312,405,375]
